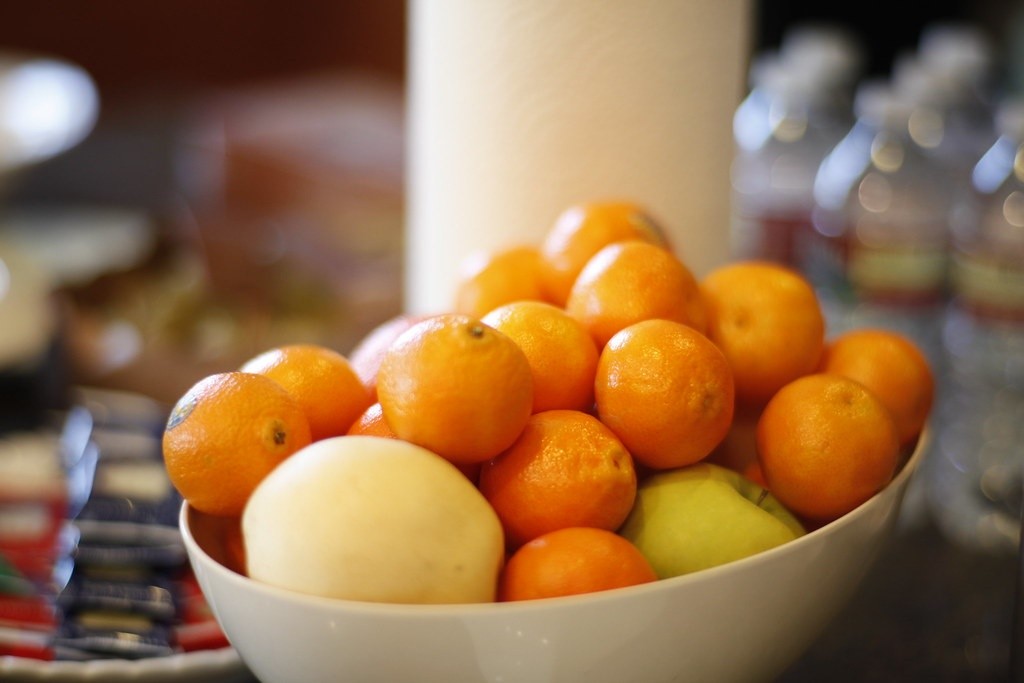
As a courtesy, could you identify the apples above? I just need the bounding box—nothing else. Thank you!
[619,463,809,579]
[239,435,505,608]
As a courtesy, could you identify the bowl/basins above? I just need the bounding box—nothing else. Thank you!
[0,61,98,175]
[179,418,928,683]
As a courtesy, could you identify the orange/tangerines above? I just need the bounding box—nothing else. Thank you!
[162,201,933,600]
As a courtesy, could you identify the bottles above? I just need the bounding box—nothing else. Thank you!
[733,30,1023,543]
